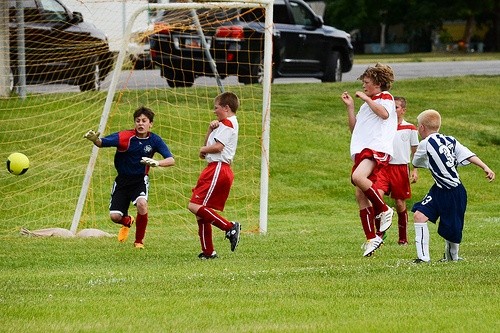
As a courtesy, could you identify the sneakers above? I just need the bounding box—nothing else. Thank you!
[362,233,384,257]
[197,250,218,260]
[224,221,242,252]
[134,240,144,249]
[118,215,134,242]
[376,204,394,233]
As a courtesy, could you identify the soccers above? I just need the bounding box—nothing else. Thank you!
[6,152,30,175]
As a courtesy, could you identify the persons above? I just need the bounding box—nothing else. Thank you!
[341,61,397,257]
[187,91,242,260]
[83,107,175,250]
[411,109,496,264]
[374,96,420,246]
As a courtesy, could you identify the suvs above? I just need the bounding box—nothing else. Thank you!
[148,0,355,87]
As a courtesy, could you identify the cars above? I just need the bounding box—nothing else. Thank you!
[126,30,156,70]
[9,0,114,95]
